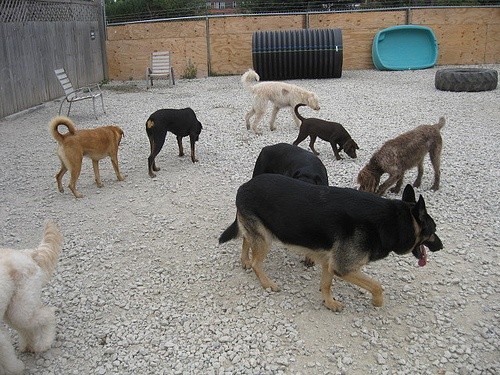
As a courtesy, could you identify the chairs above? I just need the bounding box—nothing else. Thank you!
[54,67,106,120]
[146,51,175,90]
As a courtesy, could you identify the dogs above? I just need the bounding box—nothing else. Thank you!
[219,180,445,313]
[240,70,321,132]
[250,143,329,268]
[357,118,445,194]
[48,116,124,198]
[293,103,359,160]
[146,108,202,175]
